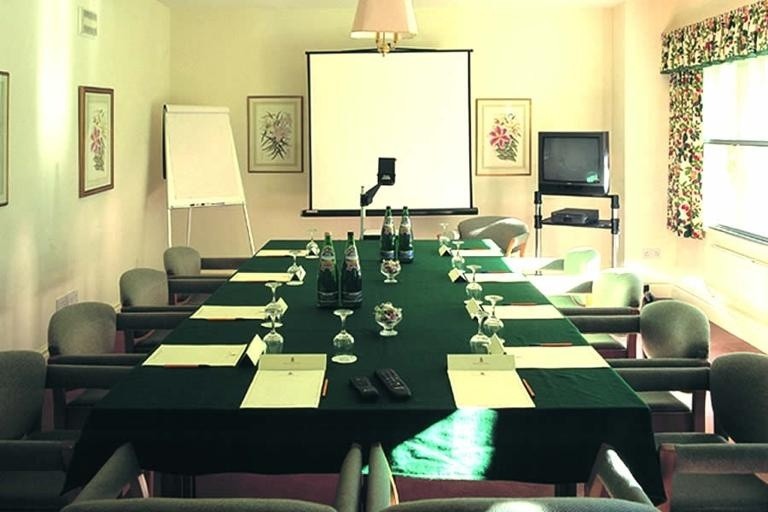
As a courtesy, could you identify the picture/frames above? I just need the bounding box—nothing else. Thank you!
[476,97,532,177]
[247,95,304,174]
[78,86,115,199]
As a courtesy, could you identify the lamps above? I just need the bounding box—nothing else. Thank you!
[348,0,417,60]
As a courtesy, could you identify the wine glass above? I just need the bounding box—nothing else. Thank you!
[258,226,319,354]
[374,304,405,338]
[379,262,402,283]
[437,221,507,353]
[330,307,355,365]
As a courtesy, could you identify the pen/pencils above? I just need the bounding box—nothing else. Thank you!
[322,378,328,396]
[522,378,535,398]
[511,302,536,305]
[208,318,232,320]
[164,365,196,368]
[540,342,573,346]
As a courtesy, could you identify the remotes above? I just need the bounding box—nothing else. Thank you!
[376,368,411,396]
[348,374,378,399]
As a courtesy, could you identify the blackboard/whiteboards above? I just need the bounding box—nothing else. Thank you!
[163,104,245,209]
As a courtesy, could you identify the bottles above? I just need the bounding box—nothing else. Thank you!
[377,205,416,264]
[316,230,363,311]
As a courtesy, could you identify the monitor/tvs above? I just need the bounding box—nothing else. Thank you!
[537,130,611,196]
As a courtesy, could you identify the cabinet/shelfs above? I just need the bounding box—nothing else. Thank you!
[535,192,619,268]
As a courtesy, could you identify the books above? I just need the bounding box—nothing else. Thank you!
[479,303,566,319]
[465,273,531,282]
[501,346,612,368]
[451,249,504,257]
[190,303,273,320]
[237,370,328,408]
[255,249,311,257]
[140,343,248,367]
[230,272,294,282]
[446,367,536,409]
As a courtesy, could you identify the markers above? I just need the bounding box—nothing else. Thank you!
[190,202,225,206]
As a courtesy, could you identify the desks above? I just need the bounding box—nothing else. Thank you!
[84,239,656,499]
